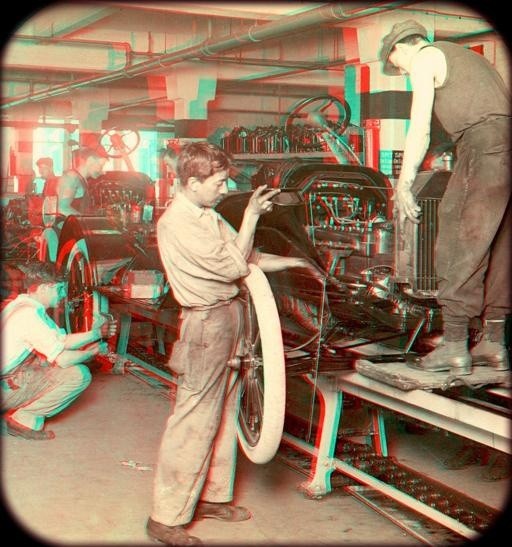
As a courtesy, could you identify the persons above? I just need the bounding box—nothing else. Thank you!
[146,138,285,546]
[375,16,511,378]
[41,144,110,264]
[1,261,122,441]
[35,155,60,197]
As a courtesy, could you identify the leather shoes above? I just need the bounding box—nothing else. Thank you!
[7,415,56,441]
[194,502,251,521]
[407,341,472,377]
[470,344,510,371]
[482,453,512,483]
[146,516,203,546]
[443,446,492,471]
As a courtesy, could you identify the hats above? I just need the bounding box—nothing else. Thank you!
[378,19,427,76]
